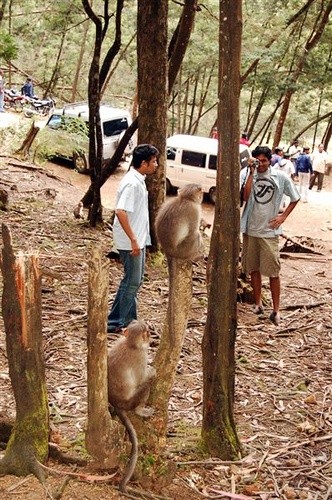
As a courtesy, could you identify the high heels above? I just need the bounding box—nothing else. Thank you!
[269,310,281,327]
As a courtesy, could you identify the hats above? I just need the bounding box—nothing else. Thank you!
[284,153,290,156]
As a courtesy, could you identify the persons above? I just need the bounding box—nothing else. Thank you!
[211,127,218,139]
[0,69,34,113]
[240,131,328,203]
[239,146,301,326]
[106,144,158,334]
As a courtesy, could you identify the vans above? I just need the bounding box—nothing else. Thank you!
[43,101,137,176]
[164,132,254,204]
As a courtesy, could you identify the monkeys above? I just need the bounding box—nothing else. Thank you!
[106,319,158,495]
[154,182,204,349]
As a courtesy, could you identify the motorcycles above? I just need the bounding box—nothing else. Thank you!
[22,95,57,117]
[2,81,25,113]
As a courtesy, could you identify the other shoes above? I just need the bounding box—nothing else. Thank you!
[302,199,308,203]
[309,187,312,190]
[316,188,321,192]
[252,303,264,314]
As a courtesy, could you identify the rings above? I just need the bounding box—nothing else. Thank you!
[276,223,278,225]
